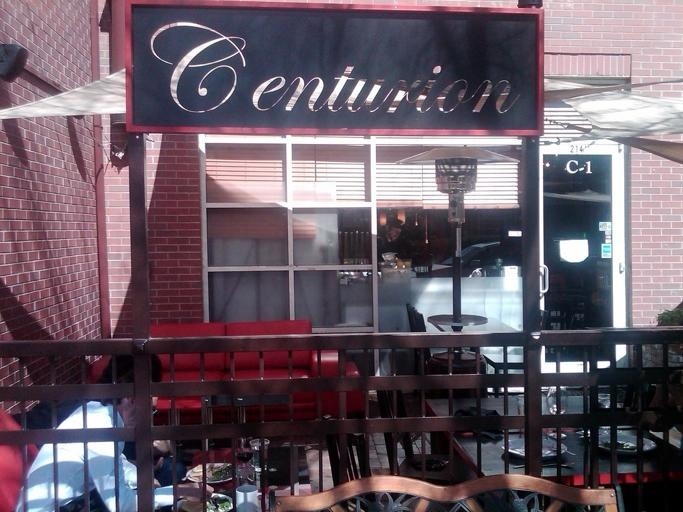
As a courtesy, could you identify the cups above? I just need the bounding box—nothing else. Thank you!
[516,395,525,416]
[249,438,270,472]
[235,485,262,512]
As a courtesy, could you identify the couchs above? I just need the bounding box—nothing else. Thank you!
[147,320,366,426]
[0,404,39,512]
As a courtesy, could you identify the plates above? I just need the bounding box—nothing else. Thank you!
[500,438,567,461]
[171,493,233,512]
[596,435,657,455]
[186,463,235,484]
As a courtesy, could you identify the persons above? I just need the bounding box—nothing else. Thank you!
[377,223,402,262]
[14,353,214,512]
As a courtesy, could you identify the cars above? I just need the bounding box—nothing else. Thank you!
[412,241,523,277]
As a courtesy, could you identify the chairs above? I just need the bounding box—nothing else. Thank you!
[325,371,486,512]
[483,310,549,397]
[405,304,462,375]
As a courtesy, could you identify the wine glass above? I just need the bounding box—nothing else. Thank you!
[234,437,254,475]
[598,393,610,430]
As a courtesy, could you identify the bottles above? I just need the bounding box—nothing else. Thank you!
[546,385,566,415]
